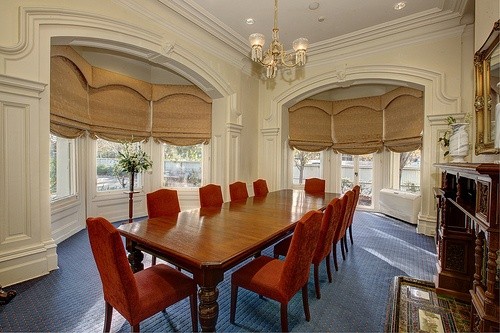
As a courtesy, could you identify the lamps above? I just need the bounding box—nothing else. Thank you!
[249,0,308,79]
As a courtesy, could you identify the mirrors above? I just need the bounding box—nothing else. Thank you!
[473,19,500,155]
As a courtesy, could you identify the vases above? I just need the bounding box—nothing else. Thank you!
[449,123,470,163]
[129,173,135,191]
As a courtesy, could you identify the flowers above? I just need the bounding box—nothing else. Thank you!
[114,134,153,174]
[438,113,473,156]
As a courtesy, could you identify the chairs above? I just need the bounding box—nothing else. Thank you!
[253,179,269,196]
[146,189,181,271]
[199,184,223,208]
[231,210,322,333]
[86,216,198,333]
[229,181,249,201]
[305,178,325,192]
[332,190,354,268]
[344,185,360,252]
[274,198,341,300]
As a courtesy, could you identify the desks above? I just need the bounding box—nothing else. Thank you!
[123,190,140,224]
[117,189,341,333]
